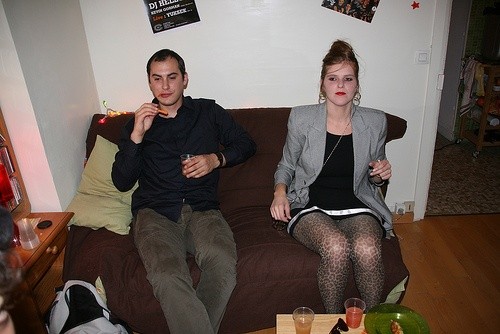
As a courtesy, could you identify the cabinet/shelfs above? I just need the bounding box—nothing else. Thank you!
[8,212,74,334]
[0,109,32,226]
[458,58,500,159]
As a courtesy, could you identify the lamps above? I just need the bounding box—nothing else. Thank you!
[0,163,20,248]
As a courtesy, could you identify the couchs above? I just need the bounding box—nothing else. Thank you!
[62,107,407,334]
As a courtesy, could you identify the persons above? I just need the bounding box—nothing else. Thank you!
[334,0,374,20]
[269,40,392,314]
[111,49,257,334]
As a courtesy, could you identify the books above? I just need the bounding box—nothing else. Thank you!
[0,134,21,212]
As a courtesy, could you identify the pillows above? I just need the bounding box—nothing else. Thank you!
[64,134,139,235]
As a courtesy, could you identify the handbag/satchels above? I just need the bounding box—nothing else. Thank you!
[41,279,134,334]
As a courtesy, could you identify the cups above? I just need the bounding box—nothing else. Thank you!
[16,218,40,250]
[180,154,196,175]
[344,298,367,329]
[292,306,315,334]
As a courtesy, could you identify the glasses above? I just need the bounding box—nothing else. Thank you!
[328,318,349,334]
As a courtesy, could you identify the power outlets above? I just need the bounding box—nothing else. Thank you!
[396,204,406,214]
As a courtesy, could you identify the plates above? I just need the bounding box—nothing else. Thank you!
[364,302,430,334]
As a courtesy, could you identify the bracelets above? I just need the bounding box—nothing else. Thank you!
[213,152,223,168]
